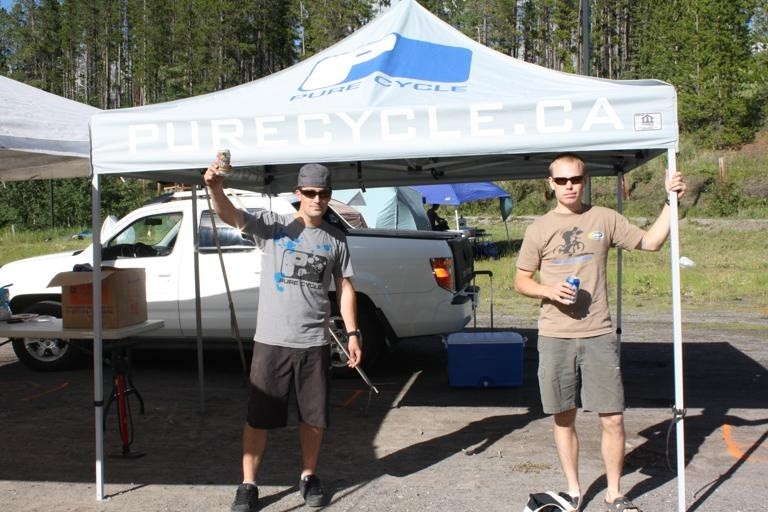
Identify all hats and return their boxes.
[291,164,337,192]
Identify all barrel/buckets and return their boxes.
[458,215,466,230]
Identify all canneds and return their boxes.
[216,149,230,177]
[565,276,580,301]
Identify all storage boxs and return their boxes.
[45,265,148,332]
[439,328,529,390]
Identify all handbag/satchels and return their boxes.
[522,490,577,512]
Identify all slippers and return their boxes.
[603,495,641,511]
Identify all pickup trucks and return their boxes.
[1,194,482,372]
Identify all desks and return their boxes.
[0,314,166,436]
[434,226,492,262]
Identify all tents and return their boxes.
[277,175,433,232]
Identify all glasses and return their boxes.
[552,175,585,185]
[297,187,333,199]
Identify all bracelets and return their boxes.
[345,329,361,339]
[665,198,681,207]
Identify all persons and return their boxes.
[458,215,466,226]
[515,151,691,512]
[427,204,449,230]
[202,151,364,512]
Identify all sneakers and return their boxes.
[298,474,324,508]
[231,481,259,512]
[567,491,583,510]
